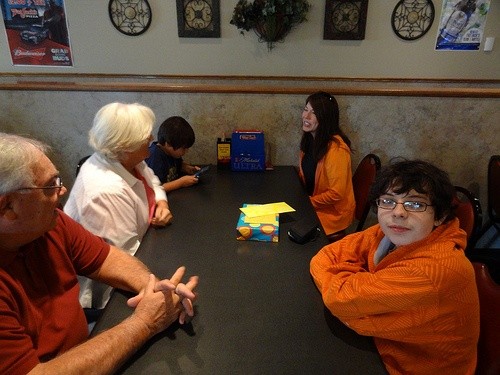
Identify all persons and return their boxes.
[309,157,480,375]
[63,102,173,336]
[300,91,358,242]
[146,116,201,188]
[0,133,198,375]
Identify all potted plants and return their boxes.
[229,0,310,48]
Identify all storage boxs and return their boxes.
[229,128,266,171]
[235,201,281,243]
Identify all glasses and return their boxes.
[19,177,62,196]
[376,197,434,212]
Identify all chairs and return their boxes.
[447,183,480,250]
[353,153,381,233]
[459,247,500,375]
[476,153,500,249]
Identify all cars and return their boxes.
[19,23,53,46]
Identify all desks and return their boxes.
[88,162,390,375]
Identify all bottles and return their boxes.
[220,132,226,142]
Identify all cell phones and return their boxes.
[194,165,212,178]
